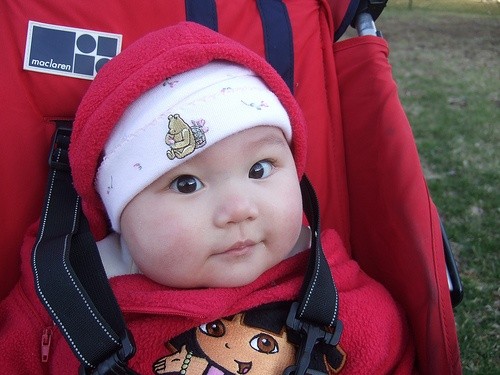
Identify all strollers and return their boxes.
[0,0,467,375]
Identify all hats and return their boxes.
[93,57,292,234]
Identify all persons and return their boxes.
[0,21,419,375]
